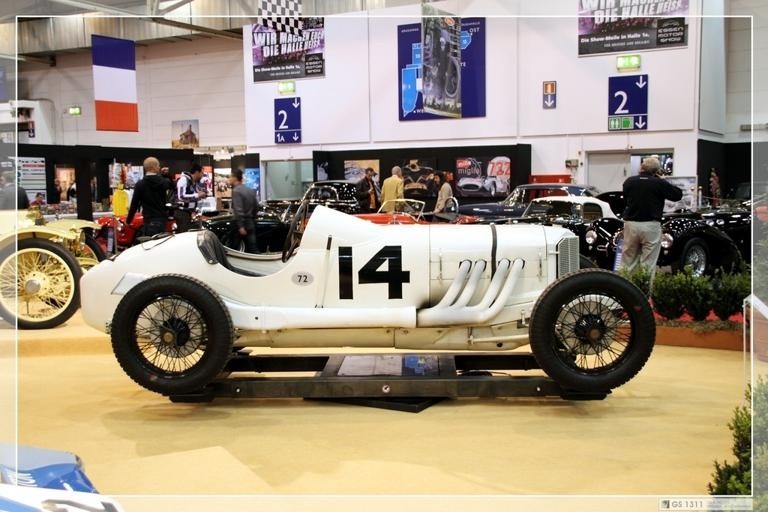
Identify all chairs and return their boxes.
[197,228,267,277]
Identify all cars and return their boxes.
[0,209,108,333]
[74,194,657,394]
[207,173,749,281]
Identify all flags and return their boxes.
[91,34,140,133]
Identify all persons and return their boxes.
[619,154,684,311]
[355,167,379,212]
[124,156,176,248]
[173,164,210,211]
[430,171,454,221]
[379,165,415,213]
[0,165,29,211]
[52,179,63,202]
[87,175,103,203]
[227,168,262,256]
[32,191,46,206]
